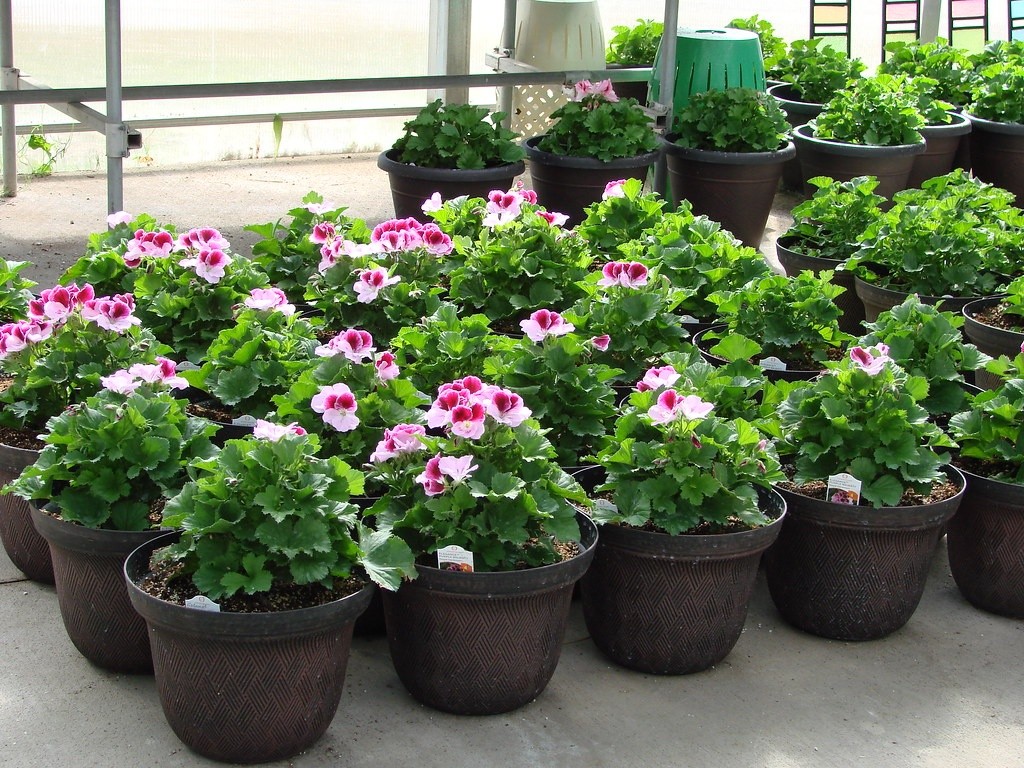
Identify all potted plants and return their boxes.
[377,16,1023,636]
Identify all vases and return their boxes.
[0,137,967,764]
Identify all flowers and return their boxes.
[0,80,948,577]
